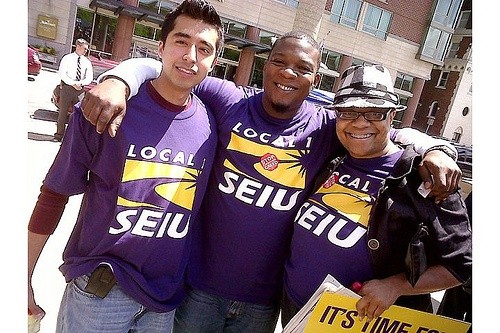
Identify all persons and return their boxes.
[52,38,93,139]
[279,61,469,333]
[29,2,221,332]
[87,29,462,332]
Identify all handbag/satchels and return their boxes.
[407,222,437,288]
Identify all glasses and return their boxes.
[334,108,393,121]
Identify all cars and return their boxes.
[28,44,42,75]
[53,53,120,112]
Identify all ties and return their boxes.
[76,57,81,81]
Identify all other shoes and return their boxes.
[53,135,64,142]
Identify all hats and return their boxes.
[323,64,408,111]
